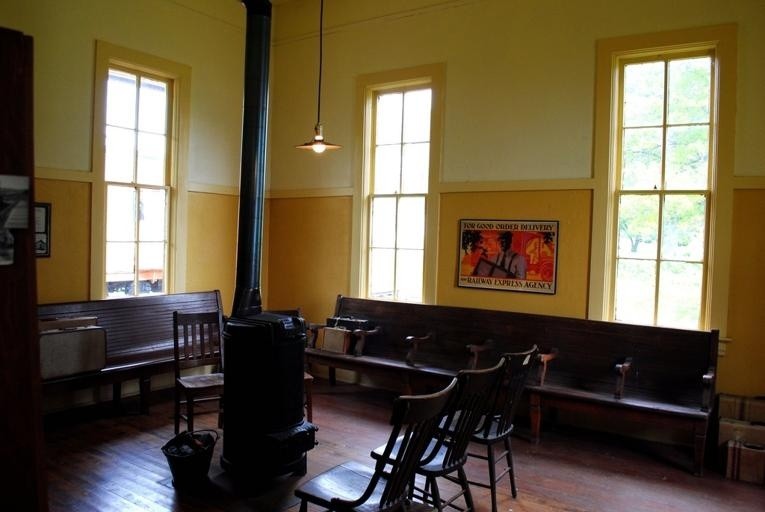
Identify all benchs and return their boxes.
[34,290,228,424]
[306,290,723,476]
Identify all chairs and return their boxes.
[265,305,315,425]
[294,343,540,510]
[170,308,228,435]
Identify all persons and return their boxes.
[490,232,528,279]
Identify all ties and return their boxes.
[500,254,506,269]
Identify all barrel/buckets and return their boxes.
[161,428,220,496]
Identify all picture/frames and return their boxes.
[34,202,52,257]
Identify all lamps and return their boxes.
[295,0,343,153]
